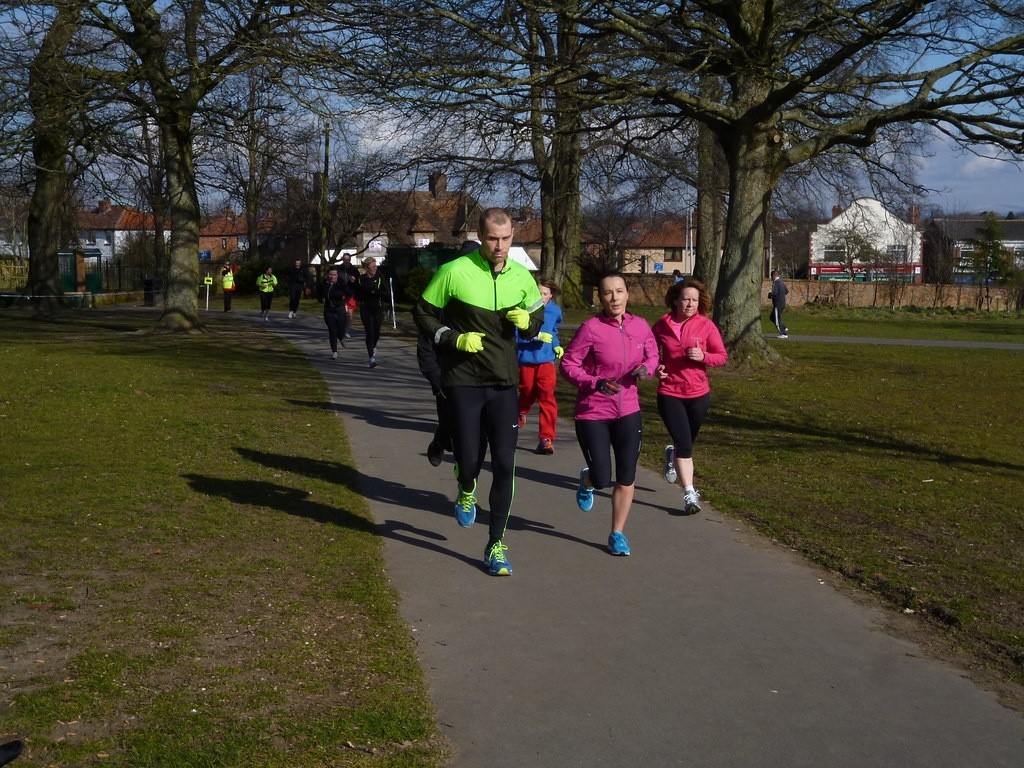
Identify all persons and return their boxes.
[336,252,359,339]
[670,269,682,283]
[257,266,278,321]
[316,266,349,360]
[412,207,545,574]
[650,278,728,514]
[559,272,659,556]
[768,269,790,339]
[218,261,235,314]
[353,257,390,369]
[282,258,312,318]
[515,274,564,455]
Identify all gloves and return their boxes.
[630,364,648,381]
[456,330,486,353]
[596,375,620,396]
[505,306,530,330]
[555,346,564,359]
[540,331,552,344]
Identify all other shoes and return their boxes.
[332,352,338,359]
[340,339,347,347]
[265,317,269,322]
[428,428,444,466]
[369,357,377,367]
[373,347,377,356]
[287,312,296,318]
[345,333,350,338]
[226,309,233,313]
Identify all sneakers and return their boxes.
[518,414,526,429]
[576,467,595,512]
[683,490,701,515]
[537,439,554,455]
[454,479,476,528]
[484,540,512,576]
[607,531,630,556]
[664,445,677,484]
